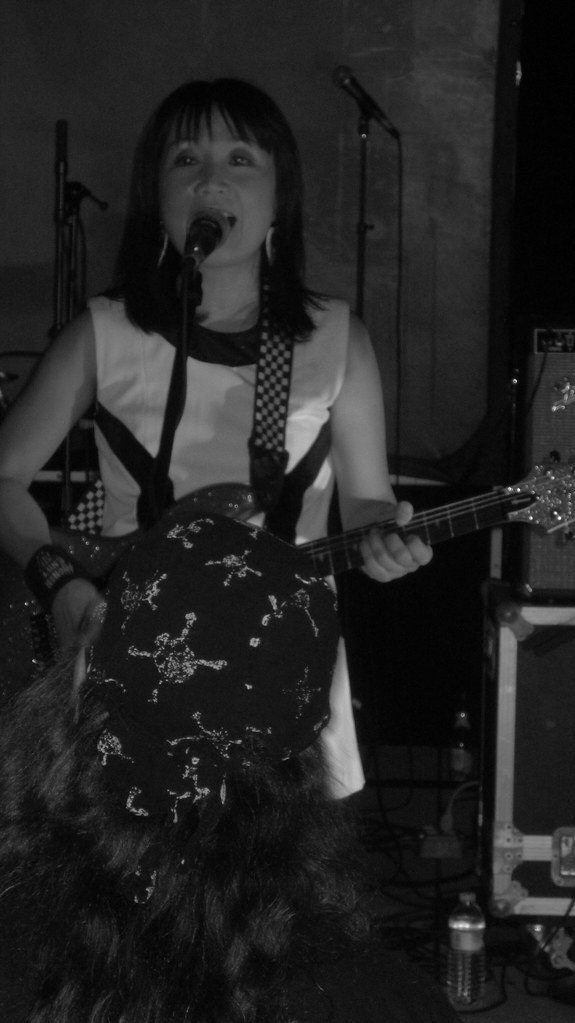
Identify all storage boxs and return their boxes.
[484,601,575,922]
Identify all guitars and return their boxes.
[0,443,574,719]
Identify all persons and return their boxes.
[0,510,460,1021]
[0,77,436,802]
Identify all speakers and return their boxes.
[513,323,575,606]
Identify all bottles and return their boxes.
[446,892,487,1007]
[448,691,474,785]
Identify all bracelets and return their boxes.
[23,541,85,612]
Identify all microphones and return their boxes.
[334,64,400,141]
[182,207,231,269]
